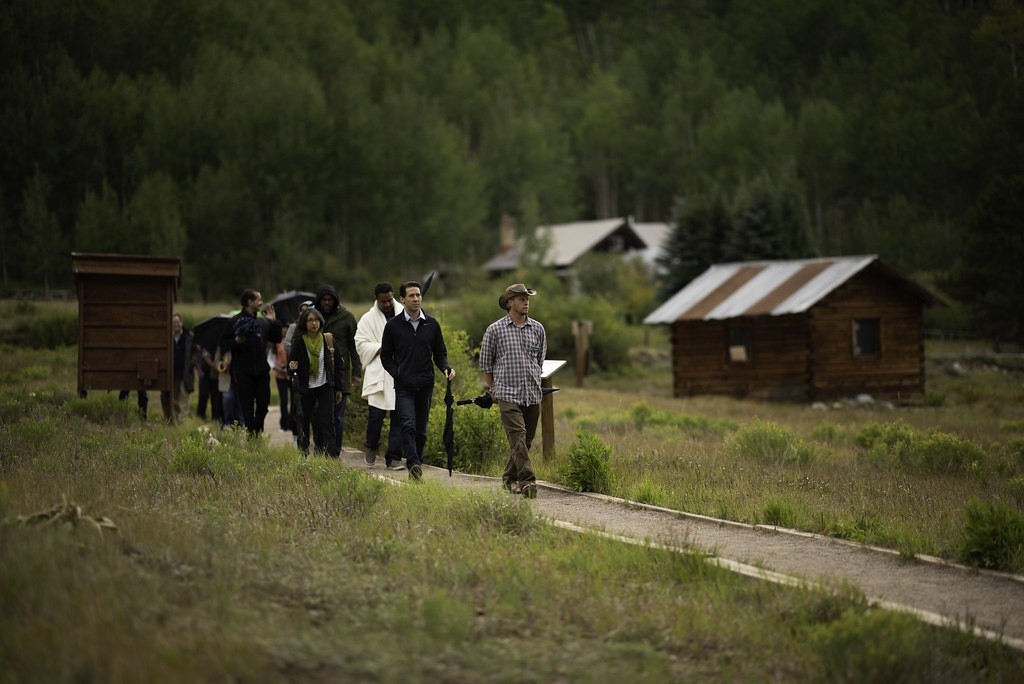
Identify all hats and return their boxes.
[498,284,537,310]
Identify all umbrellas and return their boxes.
[261,290,317,326]
[444,369,454,478]
[457,388,561,408]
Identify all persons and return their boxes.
[354,284,404,471]
[173,284,358,459]
[380,281,455,484]
[479,283,548,498]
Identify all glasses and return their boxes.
[306,316,320,323]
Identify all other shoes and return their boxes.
[409,465,422,481]
[502,480,521,493]
[387,460,405,470]
[522,483,537,499]
[364,447,376,465]
